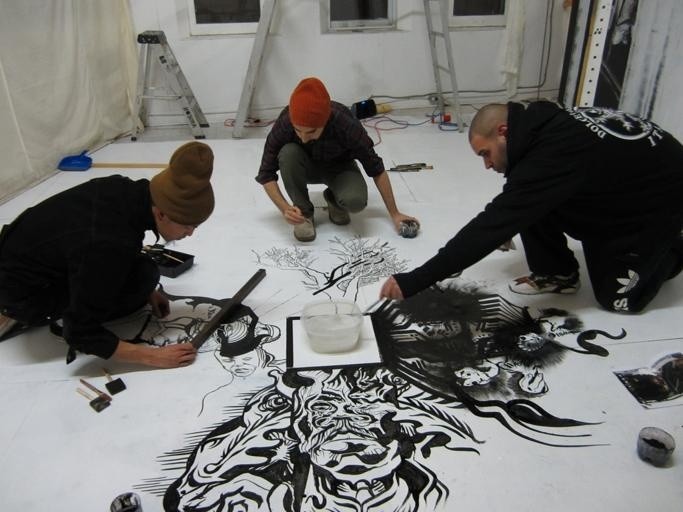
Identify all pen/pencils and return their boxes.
[103,368,126,396]
[77,388,111,412]
[289,206,310,224]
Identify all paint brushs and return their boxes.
[386,163,433,172]
[80,379,112,401]
[361,297,382,316]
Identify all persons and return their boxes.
[0,176,214,370]
[378,98,682,318]
[254,76,421,242]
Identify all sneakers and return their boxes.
[323,188,350,225]
[294,217,316,242]
[508,272,580,295]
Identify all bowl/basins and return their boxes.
[304,300,363,353]
[637,426,674,466]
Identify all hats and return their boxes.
[290,78,331,128]
[151,142,214,226]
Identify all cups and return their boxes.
[109,492,141,511]
[399,218,419,237]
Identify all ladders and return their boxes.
[131,31,210,141]
[232,0,277,138]
[422,1,463,131]
